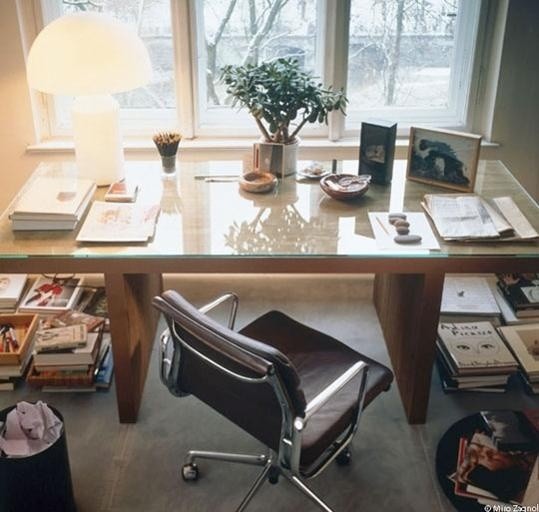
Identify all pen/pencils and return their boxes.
[195,175,241,182]
[0,324,19,353]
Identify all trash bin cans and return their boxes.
[0,402,76,510]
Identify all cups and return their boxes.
[163,175,178,198]
[158,156,178,174]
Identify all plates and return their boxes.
[298,168,333,179]
[294,177,321,184]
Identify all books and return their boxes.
[9,171,162,249]
[431,267,538,511]
[0,270,113,397]
[424,187,539,248]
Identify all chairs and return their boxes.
[153,290,395,512]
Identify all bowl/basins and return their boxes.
[318,196,373,217]
[318,173,370,201]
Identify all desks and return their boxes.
[3,161,537,425]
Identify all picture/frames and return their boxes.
[406,126,482,194]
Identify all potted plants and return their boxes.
[221,60,348,175]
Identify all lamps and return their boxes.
[25,11,151,186]
